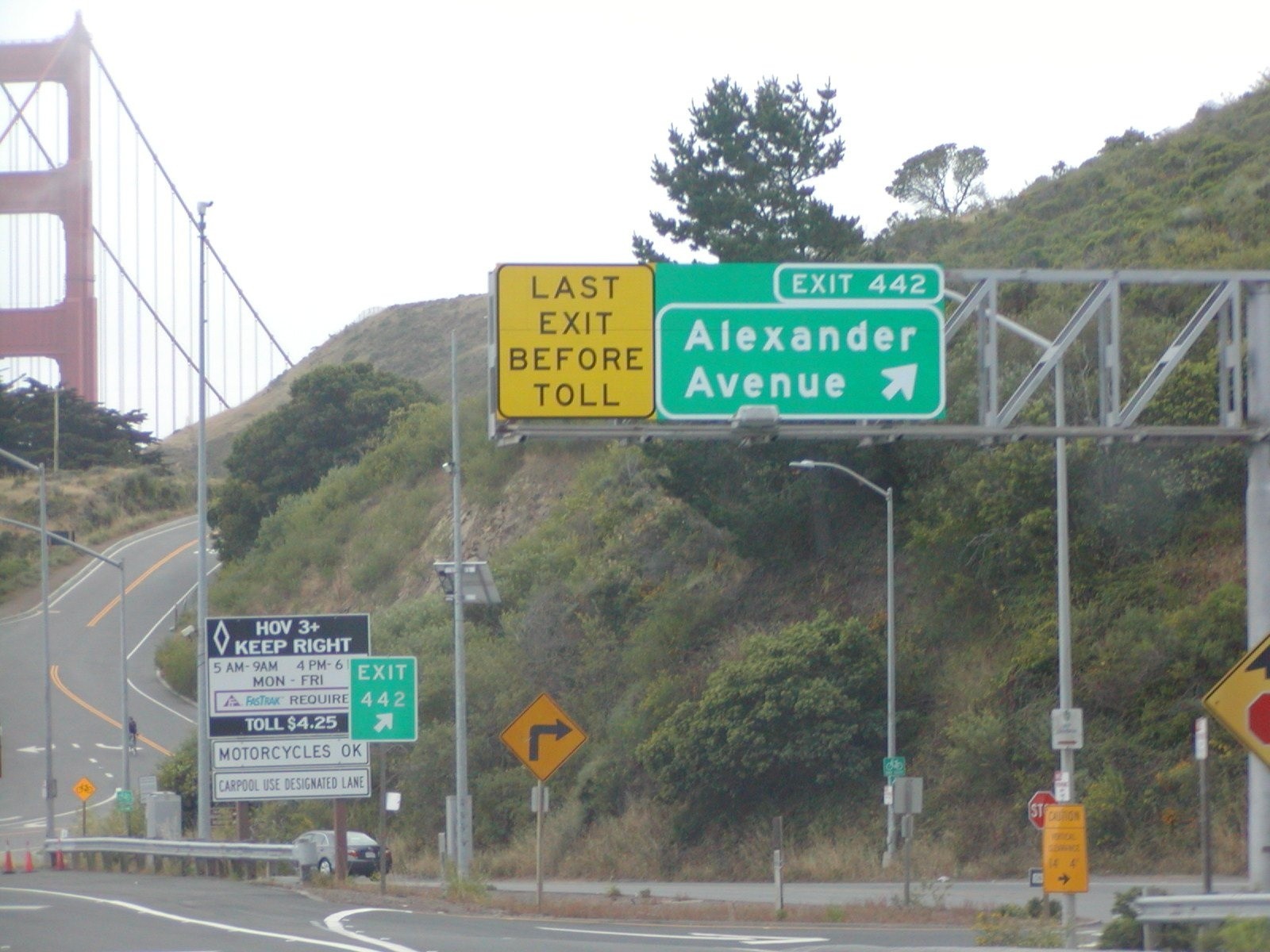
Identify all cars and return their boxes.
[290,829,393,882]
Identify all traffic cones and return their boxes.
[22,840,37,873]
[52,851,67,873]
[2,840,18,874]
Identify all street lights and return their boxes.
[787,459,899,870]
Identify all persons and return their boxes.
[128,716,138,747]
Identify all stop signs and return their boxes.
[1028,791,1058,830]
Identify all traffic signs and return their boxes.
[348,655,419,743]
[493,262,947,421]
